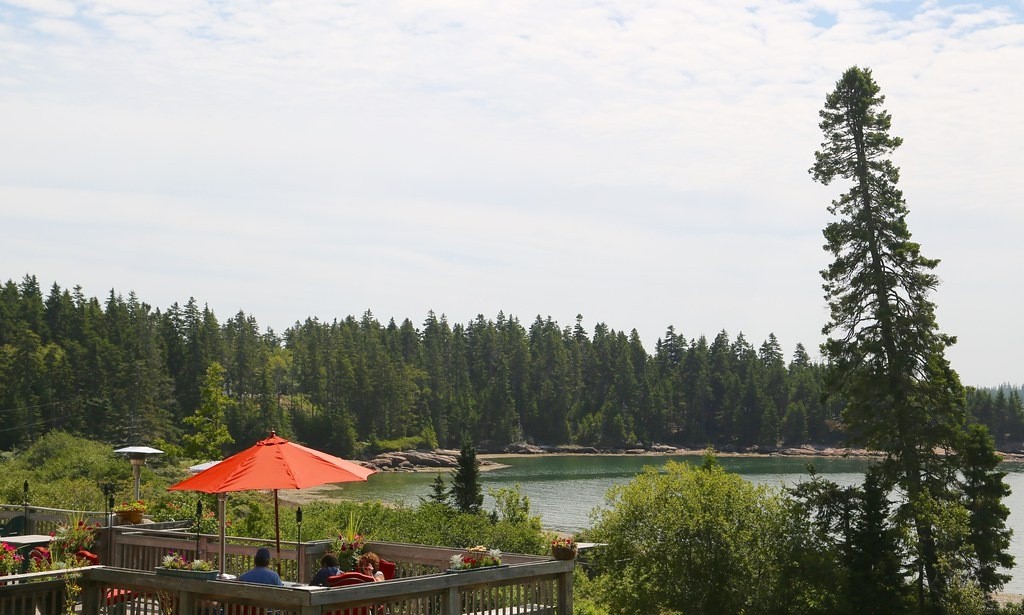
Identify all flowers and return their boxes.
[552,537,576,548]
[162,550,216,571]
[0,542,23,573]
[45,506,97,555]
[328,509,382,571]
[449,545,503,569]
[111,500,147,512]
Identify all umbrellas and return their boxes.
[167,429,376,579]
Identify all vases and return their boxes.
[551,547,576,560]
[447,563,509,573]
[154,567,219,580]
[0,573,8,587]
[114,511,145,525]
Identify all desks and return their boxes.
[576,543,611,550]
[0,534,58,583]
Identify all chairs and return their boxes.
[328,572,375,615]
[73,551,138,606]
[0,516,35,537]
[370,559,394,613]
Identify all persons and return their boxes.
[357,552,396,580]
[309,555,342,587]
[239,548,282,585]
